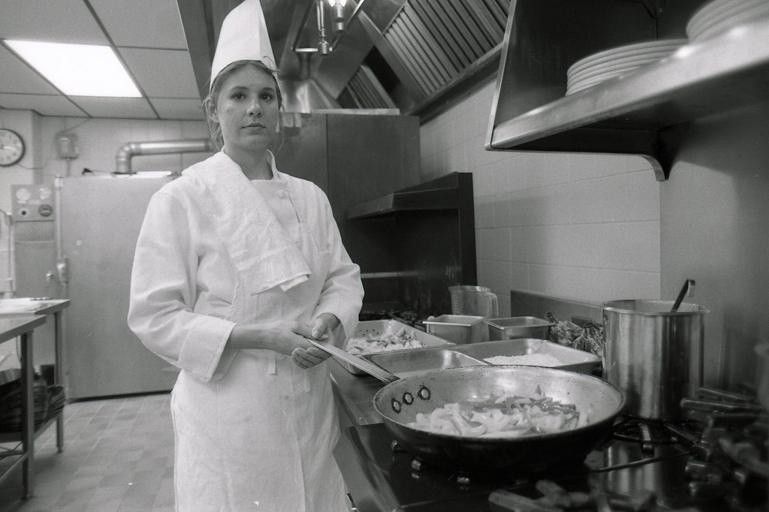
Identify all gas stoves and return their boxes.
[331,386,769,512]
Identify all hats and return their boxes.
[209,0,283,105]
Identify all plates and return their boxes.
[564,0,768,100]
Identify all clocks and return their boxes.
[0,129,24,166]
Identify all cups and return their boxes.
[450,284,502,319]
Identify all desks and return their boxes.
[1,298,70,466]
[1,314,48,500]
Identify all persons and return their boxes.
[127,1,367,512]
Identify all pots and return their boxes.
[603,298,712,422]
[302,336,628,466]
